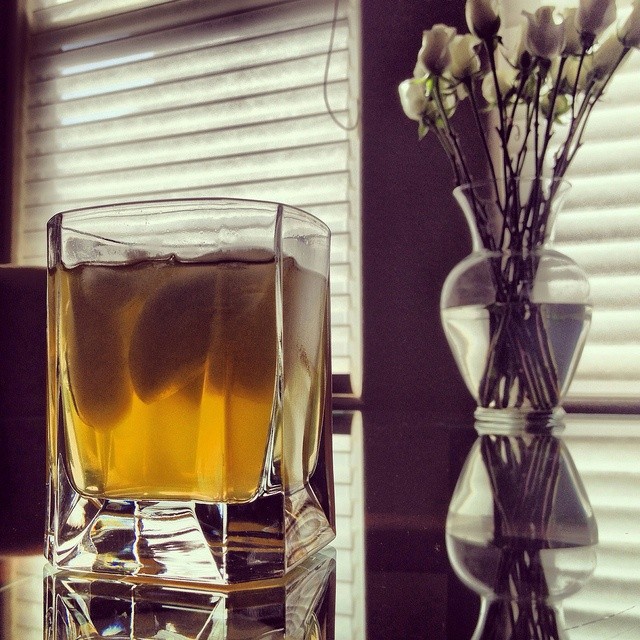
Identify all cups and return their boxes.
[43,198,336,593]
[42,547,336,639]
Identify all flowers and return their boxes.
[398,0,640,410]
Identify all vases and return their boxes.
[445,422,600,639]
[441,178,593,424]
